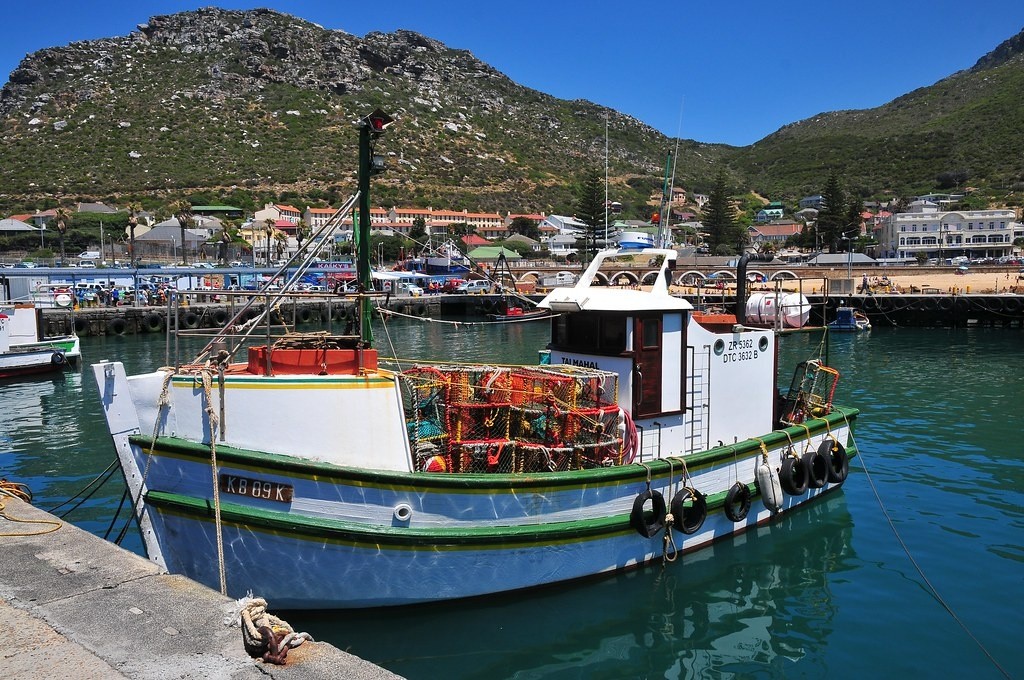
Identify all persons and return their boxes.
[858,272,871,295]
[68,277,472,310]
[591,279,642,291]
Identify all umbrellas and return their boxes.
[247,275,269,290]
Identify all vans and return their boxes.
[77,251,101,259]
[443,278,491,294]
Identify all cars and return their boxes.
[396,283,425,296]
[4,260,95,268]
[929,255,1024,263]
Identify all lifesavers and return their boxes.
[108,318,130,336]
[632,490,667,540]
[682,296,1024,313]
[804,451,830,489]
[296,308,313,324]
[241,309,259,324]
[52,353,65,365]
[211,310,228,327]
[271,309,284,324]
[818,439,850,483]
[725,482,751,522]
[320,298,532,323]
[671,487,708,535]
[75,318,90,336]
[165,316,181,329]
[142,312,165,333]
[181,312,201,329]
[780,458,809,496]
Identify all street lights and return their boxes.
[841,237,858,279]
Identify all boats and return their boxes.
[485,298,549,321]
[0,284,81,378]
[92,107,859,617]
[827,307,872,332]
